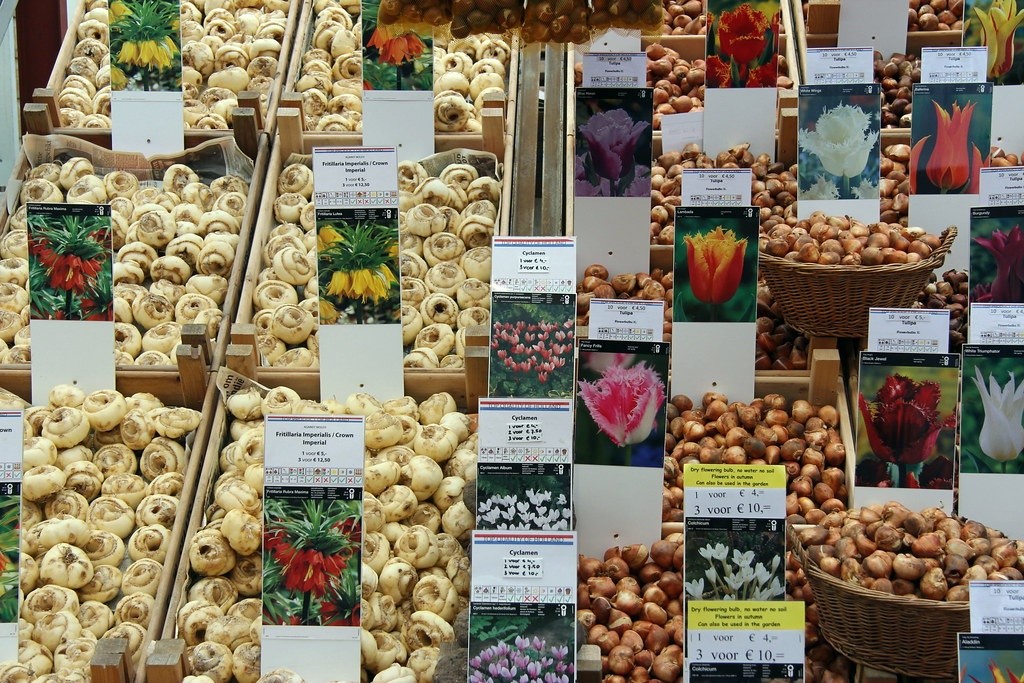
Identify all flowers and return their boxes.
[675,223,757,322]
[491,318,574,384]
[361,1,427,92]
[971,224,1024,303]
[315,219,401,324]
[263,485,361,627]
[469,634,574,683]
[962,0,1024,85]
[798,100,879,200]
[108,0,184,91]
[964,364,1024,474]
[707,3,777,87]
[576,351,666,466]
[858,373,957,490]
[685,542,785,601]
[477,487,571,530]
[910,99,990,194]
[27,215,114,321]
[576,108,650,197]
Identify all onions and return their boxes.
[575,391,857,683]
[799,500,1024,601]
[378,0,663,44]
[575,0,1024,374]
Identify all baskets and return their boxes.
[788,525,971,677]
[758,225,957,338]
